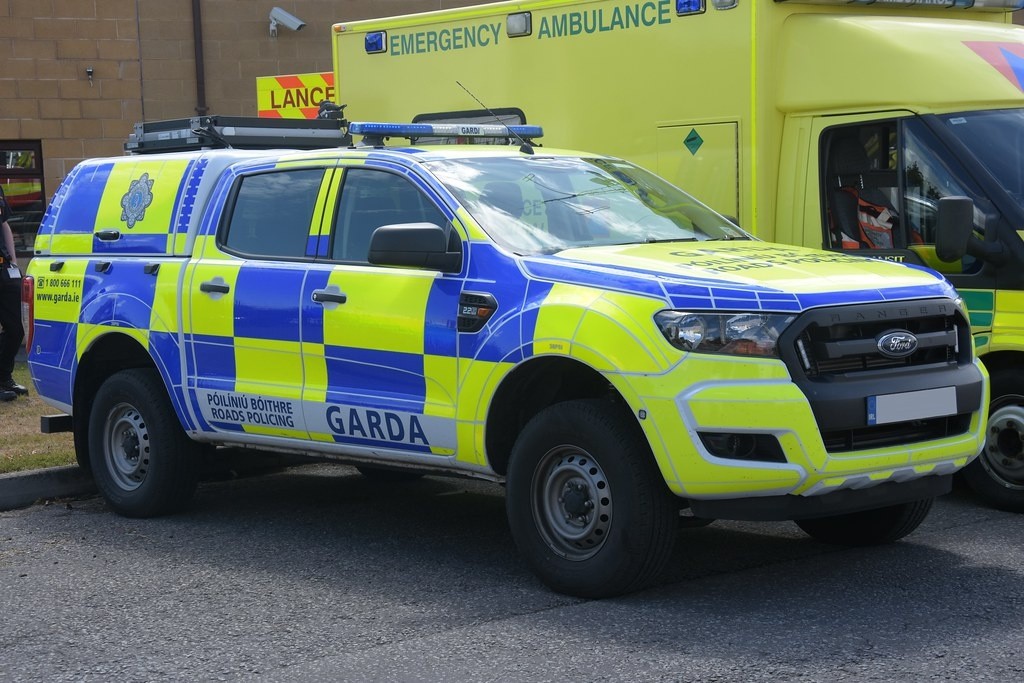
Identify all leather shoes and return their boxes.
[0,388,16,400]
[0,379,28,394]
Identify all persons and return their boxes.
[0,183,29,402]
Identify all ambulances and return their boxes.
[331,0,1024,513]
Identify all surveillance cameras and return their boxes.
[268,7,304,36]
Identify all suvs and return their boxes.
[21,100,990,600]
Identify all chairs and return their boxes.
[835,172,921,253]
